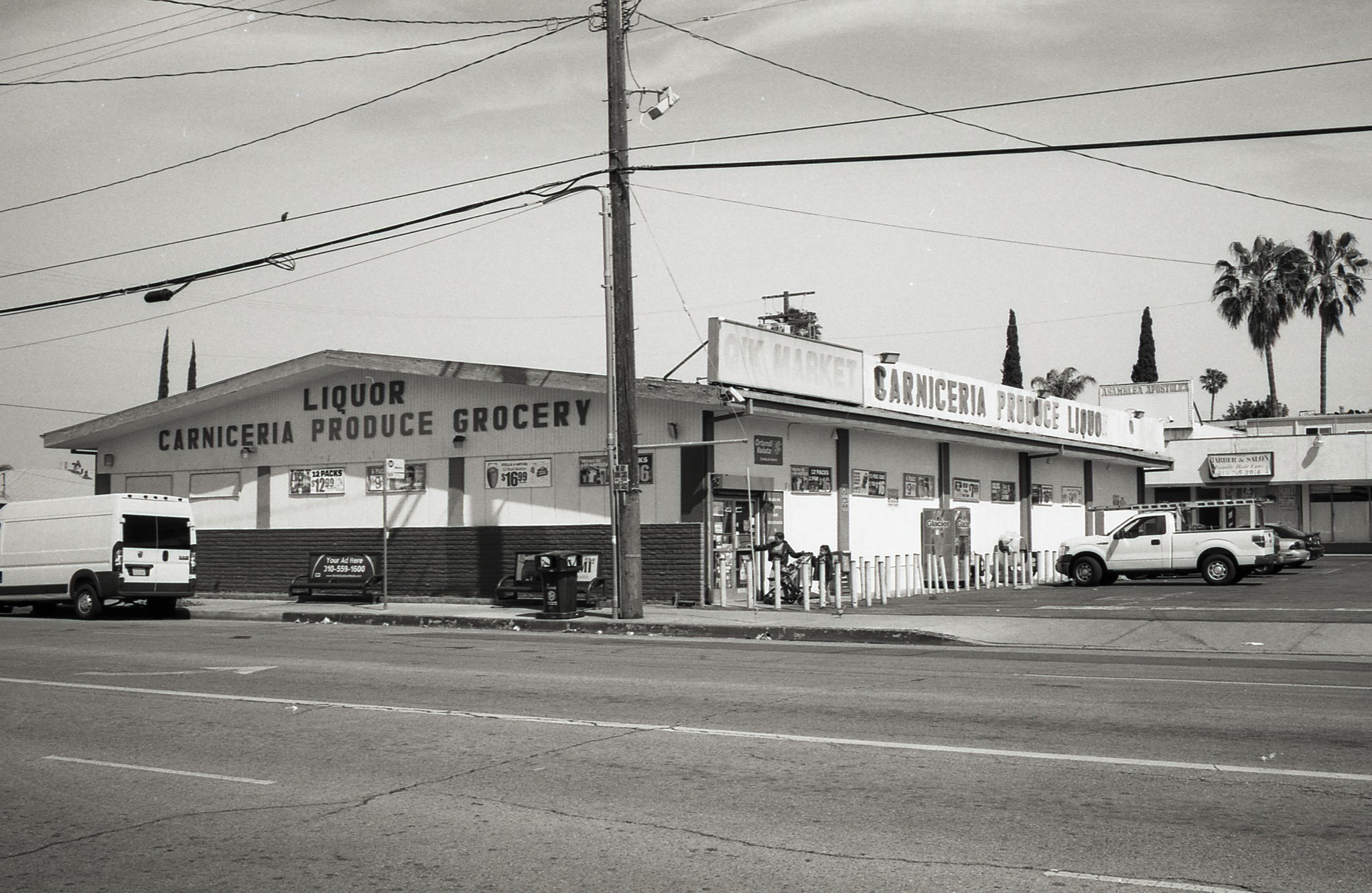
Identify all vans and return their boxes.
[0,493,198,622]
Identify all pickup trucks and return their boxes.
[1054,511,1281,588]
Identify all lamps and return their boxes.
[1046,457,1052,464]
[1037,390,1051,399]
[1313,434,1323,448]
[667,422,678,439]
[240,446,258,460]
[1132,411,1145,419]
[830,427,839,439]
[453,435,466,448]
[1106,463,1112,471]
[104,454,114,466]
[874,352,900,363]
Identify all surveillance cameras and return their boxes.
[1168,416,1174,423]
[648,93,679,120]
[726,387,745,402]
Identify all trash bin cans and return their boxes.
[535,551,584,618]
[829,551,852,596]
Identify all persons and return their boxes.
[811,545,833,606]
[745,532,805,605]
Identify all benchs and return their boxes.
[288,551,384,605]
[494,551,608,610]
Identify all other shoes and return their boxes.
[816,600,820,604]
[826,601,831,606]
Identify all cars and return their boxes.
[1236,522,1325,567]
[1253,536,1311,575]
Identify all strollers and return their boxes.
[764,552,813,605]
